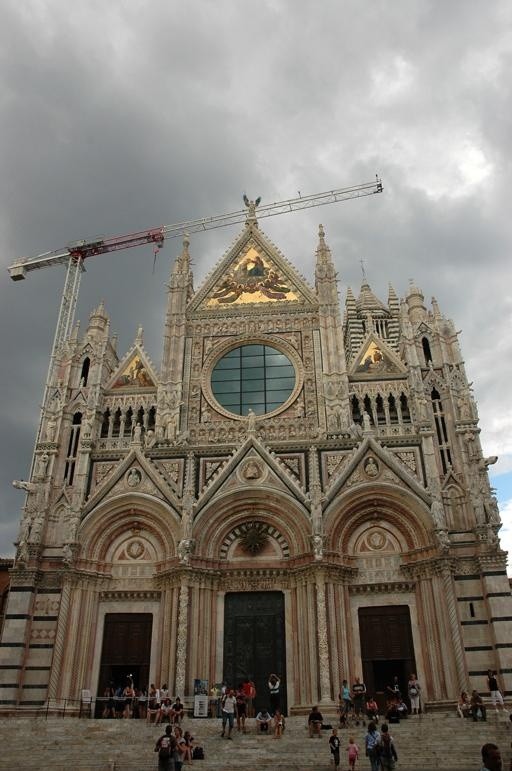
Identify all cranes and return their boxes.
[5,173,384,570]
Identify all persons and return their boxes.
[100,681,185,725]
[328,726,342,766]
[154,725,184,771]
[345,737,360,771]
[479,742,503,771]
[169,726,186,771]
[182,730,194,764]
[365,722,381,771]
[376,723,399,771]
[268,673,281,716]
[485,669,509,713]
[385,675,422,722]
[193,684,208,697]
[308,706,325,738]
[210,676,255,740]
[337,676,378,726]
[256,709,285,739]
[457,690,488,723]
